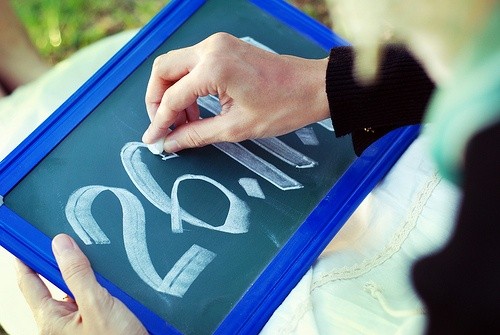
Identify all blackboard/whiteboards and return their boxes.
[0,0,428,335]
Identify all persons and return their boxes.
[0,7,499,335]
